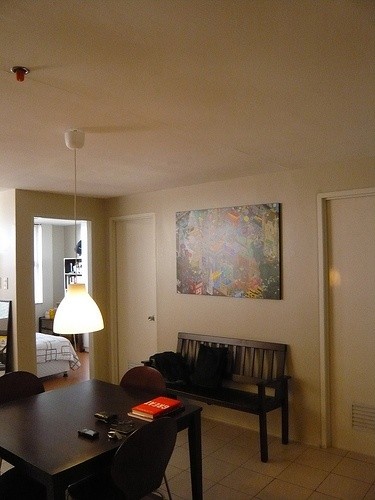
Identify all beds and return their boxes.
[36,331,82,378]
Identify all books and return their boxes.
[65,275,76,289]
[127,395,183,422]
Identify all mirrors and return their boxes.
[0,300,12,376]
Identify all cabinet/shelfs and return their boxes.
[39,316,73,352]
[64,258,89,353]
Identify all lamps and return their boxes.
[53,128,105,335]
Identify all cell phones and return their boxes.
[95,412,117,419]
[110,425,134,434]
[78,429,99,439]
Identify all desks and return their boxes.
[0,379,203,500]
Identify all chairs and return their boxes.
[0,371,46,404]
[120,367,167,398]
[64,416,177,500]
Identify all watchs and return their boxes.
[107,431,122,440]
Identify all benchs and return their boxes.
[140,333,292,462]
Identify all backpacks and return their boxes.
[189,344,235,397]
[149,351,193,391]
